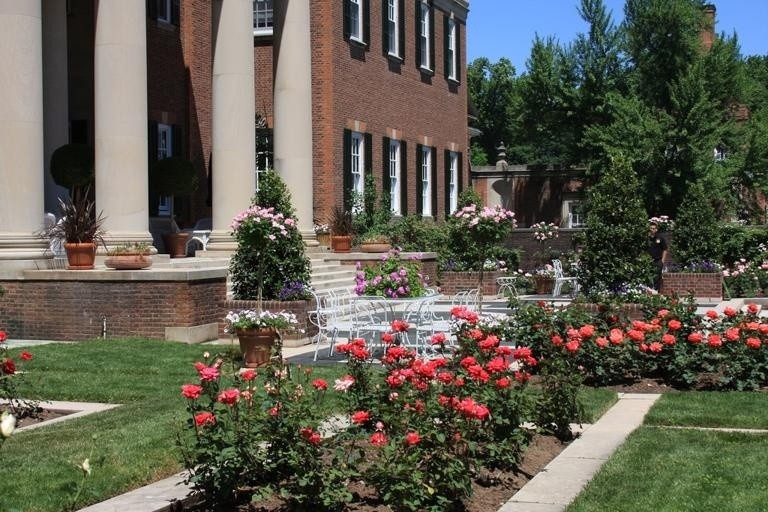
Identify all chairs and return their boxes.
[306,287,479,362]
[552,258,579,298]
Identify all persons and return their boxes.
[645,221,668,293]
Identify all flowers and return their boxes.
[220,306,305,348]
[352,244,431,297]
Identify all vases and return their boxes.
[236,324,276,369]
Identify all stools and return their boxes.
[496,276,518,299]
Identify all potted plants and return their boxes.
[35,182,156,270]
